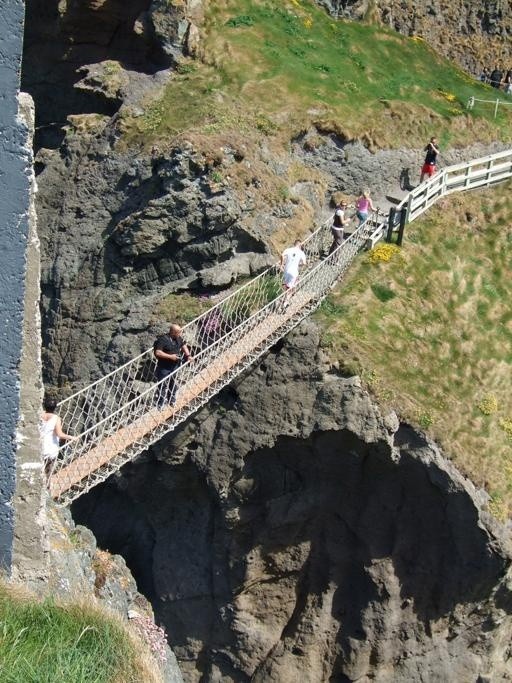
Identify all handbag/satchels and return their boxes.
[185,354,191,358]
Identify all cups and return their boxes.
[341,204,347,207]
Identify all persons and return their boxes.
[356,188,384,228]
[330,200,351,253]
[479,63,512,93]
[42,398,77,489]
[277,239,307,315]
[420,137,440,183]
[154,324,194,412]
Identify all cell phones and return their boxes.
[178,355,183,359]
[428,144,431,147]
